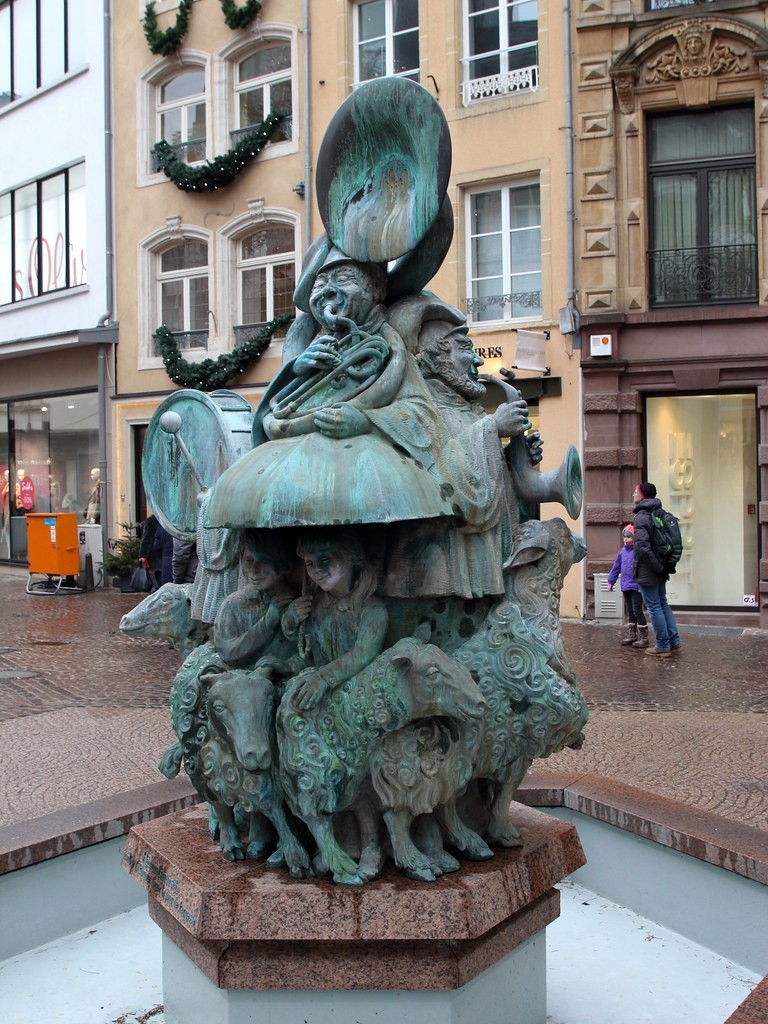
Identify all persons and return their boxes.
[2,470,29,560]
[606,524,649,648]
[632,482,683,658]
[85,469,101,524]
[138,514,199,586]
[160,248,586,883]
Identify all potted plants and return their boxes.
[96,520,146,591]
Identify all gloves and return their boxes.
[663,570,670,581]
[664,561,677,574]
[607,583,613,591]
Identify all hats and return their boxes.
[638,483,657,499]
[622,524,634,538]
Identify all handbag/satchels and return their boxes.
[130,560,153,593]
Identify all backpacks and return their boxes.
[651,508,683,563]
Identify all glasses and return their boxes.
[623,535,631,538]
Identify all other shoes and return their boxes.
[670,645,683,653]
[645,647,670,657]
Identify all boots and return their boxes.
[621,623,638,645]
[632,624,650,647]
